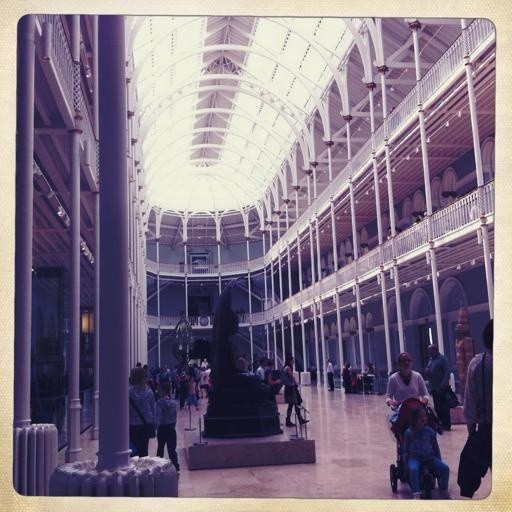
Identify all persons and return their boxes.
[326,358,334,391]
[341,360,376,395]
[178,307,246,323]
[385,318,493,500]
[129,353,310,474]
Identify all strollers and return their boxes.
[385,397,442,496]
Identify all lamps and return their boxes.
[80,305,94,343]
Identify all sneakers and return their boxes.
[284,415,310,427]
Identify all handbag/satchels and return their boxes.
[430,385,461,414]
[142,422,157,439]
